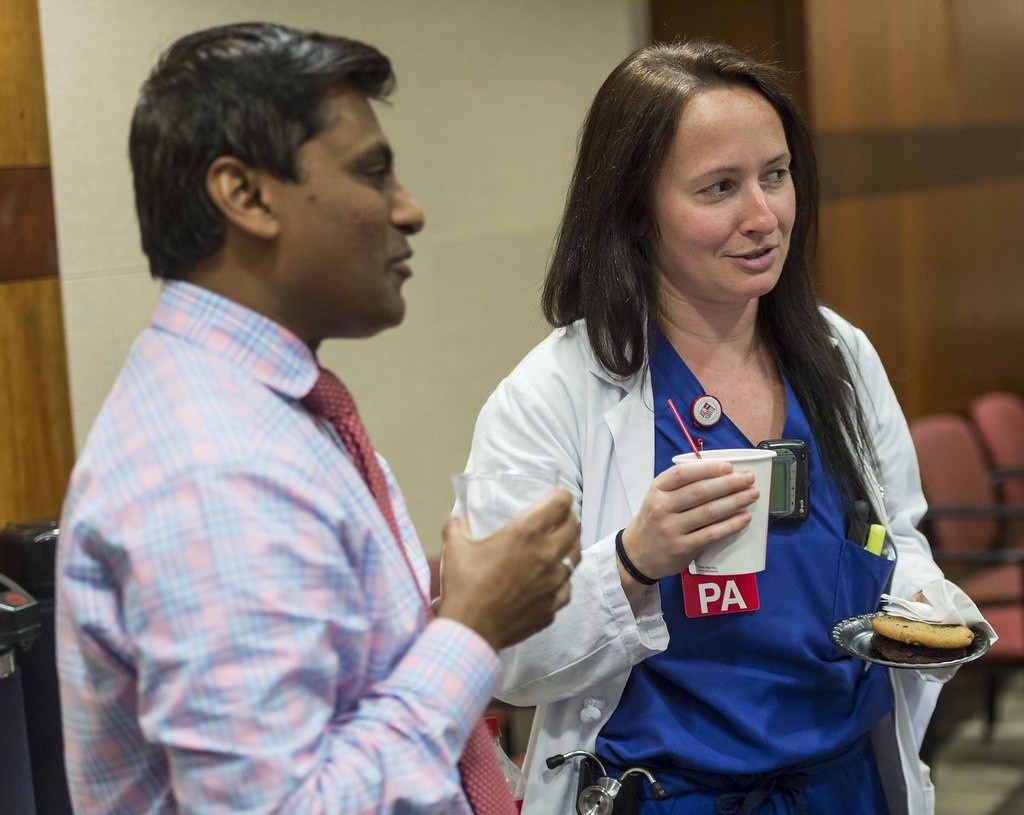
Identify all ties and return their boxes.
[300,366,518,815]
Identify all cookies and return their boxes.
[871,614,975,648]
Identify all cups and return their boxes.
[452,469,564,545]
[672,448,776,576]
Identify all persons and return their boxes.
[441,39,999,815]
[55,23,583,814]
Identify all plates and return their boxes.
[834,612,989,669]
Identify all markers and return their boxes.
[846,496,888,556]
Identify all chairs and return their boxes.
[909,392,1024,749]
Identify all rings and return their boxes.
[561,556,575,573]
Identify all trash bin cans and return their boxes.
[0,520,75,815]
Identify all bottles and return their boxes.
[484,716,527,815]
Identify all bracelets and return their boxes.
[616,528,662,585]
